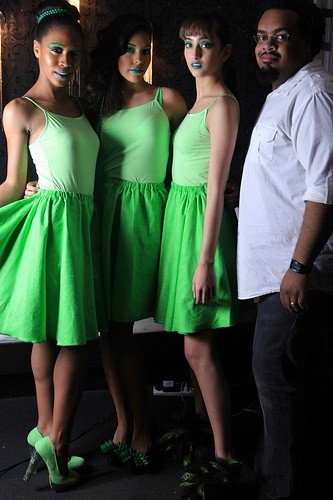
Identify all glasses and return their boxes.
[253,33,301,43]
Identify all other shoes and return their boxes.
[179,449,244,500]
[151,414,215,469]
[100,433,155,475]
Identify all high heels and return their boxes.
[22,423,86,491]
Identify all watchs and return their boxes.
[289,259,314,275]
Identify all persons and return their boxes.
[153,15,240,482]
[237,0,333,500]
[0,7,100,491]
[23,13,240,475]
